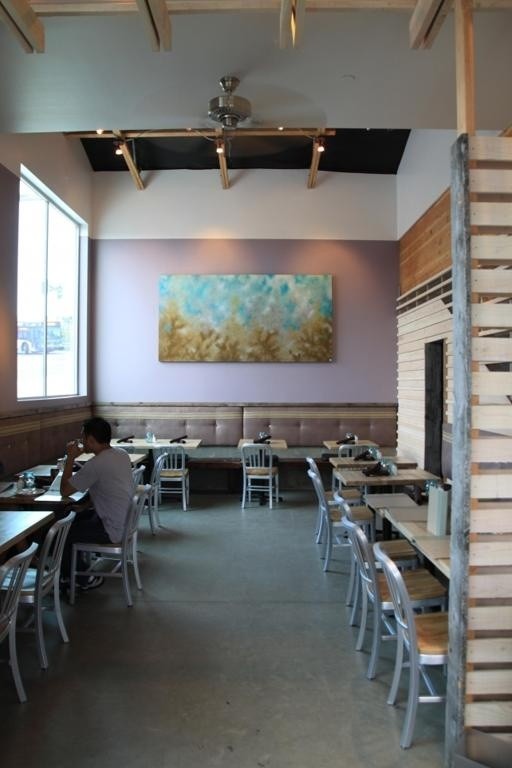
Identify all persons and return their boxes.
[25,416,137,600]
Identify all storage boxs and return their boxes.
[427,484,452,538]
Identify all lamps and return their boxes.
[113,140,124,156]
[314,137,326,151]
[214,139,224,153]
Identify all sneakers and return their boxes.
[60,571,79,584]
[82,573,104,590]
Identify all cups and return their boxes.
[257,432,268,439]
[379,458,397,475]
[23,472,35,490]
[76,438,83,449]
[146,432,154,443]
[345,432,358,443]
[425,479,438,502]
[368,448,381,460]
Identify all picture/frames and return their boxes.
[159,274,334,362]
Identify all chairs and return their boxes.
[334,492,418,627]
[241,443,279,510]
[135,454,168,537]
[306,457,362,534]
[69,483,152,607]
[132,465,146,485]
[341,516,447,681]
[308,469,375,572]
[373,542,448,750]
[0,542,39,703]
[154,446,189,511]
[0,511,77,673]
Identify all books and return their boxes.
[34,471,90,502]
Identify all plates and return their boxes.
[16,488,46,496]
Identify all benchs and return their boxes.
[121,448,397,494]
